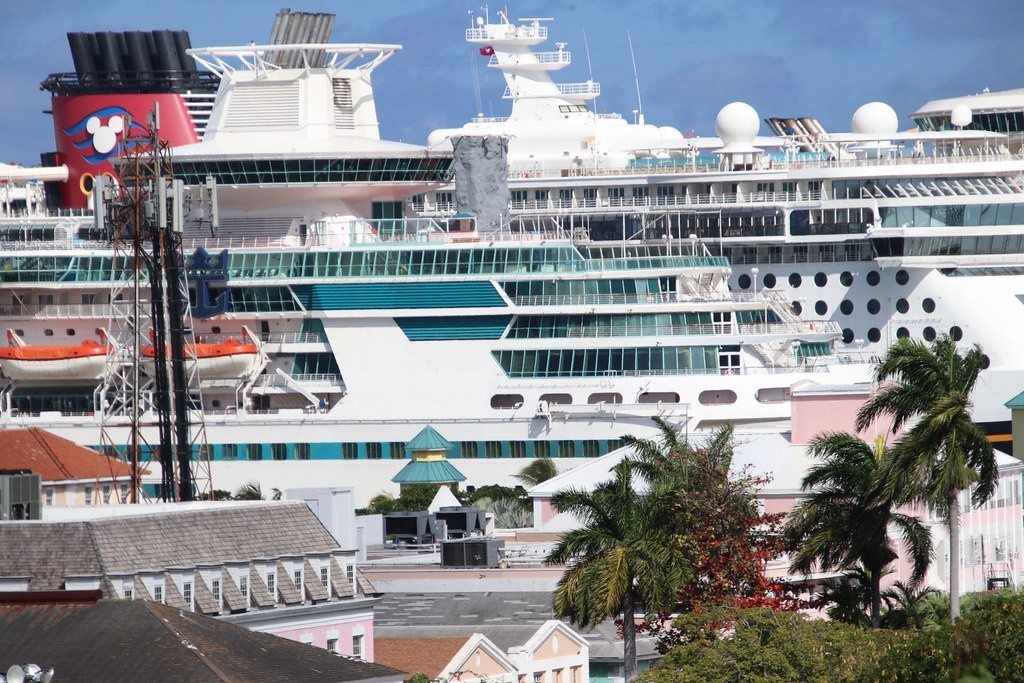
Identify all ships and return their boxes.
[0,0,1024,517]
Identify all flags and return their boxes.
[479,46,495,55]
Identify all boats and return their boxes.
[1,337,114,382]
[138,338,257,381]
